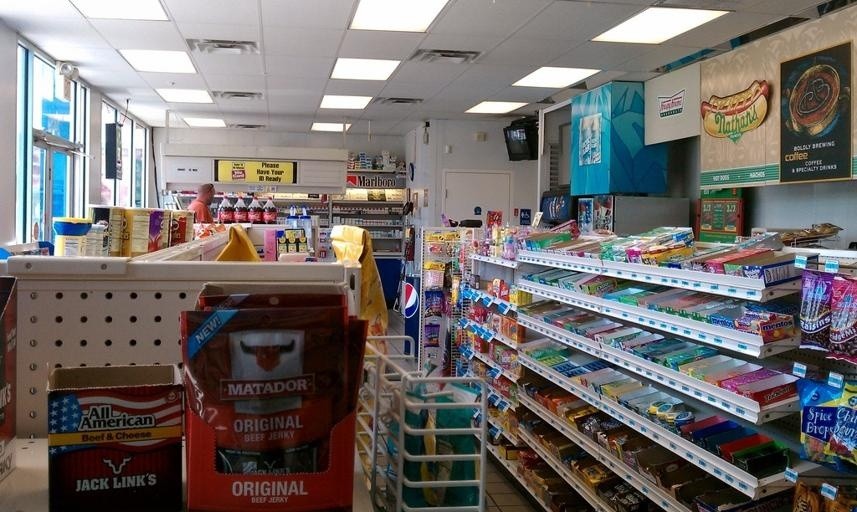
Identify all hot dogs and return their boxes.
[701,80,770,137]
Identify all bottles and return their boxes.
[217,196,277,224]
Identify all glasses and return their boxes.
[207,184,213,194]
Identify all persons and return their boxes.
[187,184,215,223]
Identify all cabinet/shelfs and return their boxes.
[173,194,857,512]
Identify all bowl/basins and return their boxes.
[52,217,91,235]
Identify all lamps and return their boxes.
[54,61,80,103]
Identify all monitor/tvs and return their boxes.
[540,190,577,225]
[503,117,538,161]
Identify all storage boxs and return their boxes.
[46,364,183,512]
